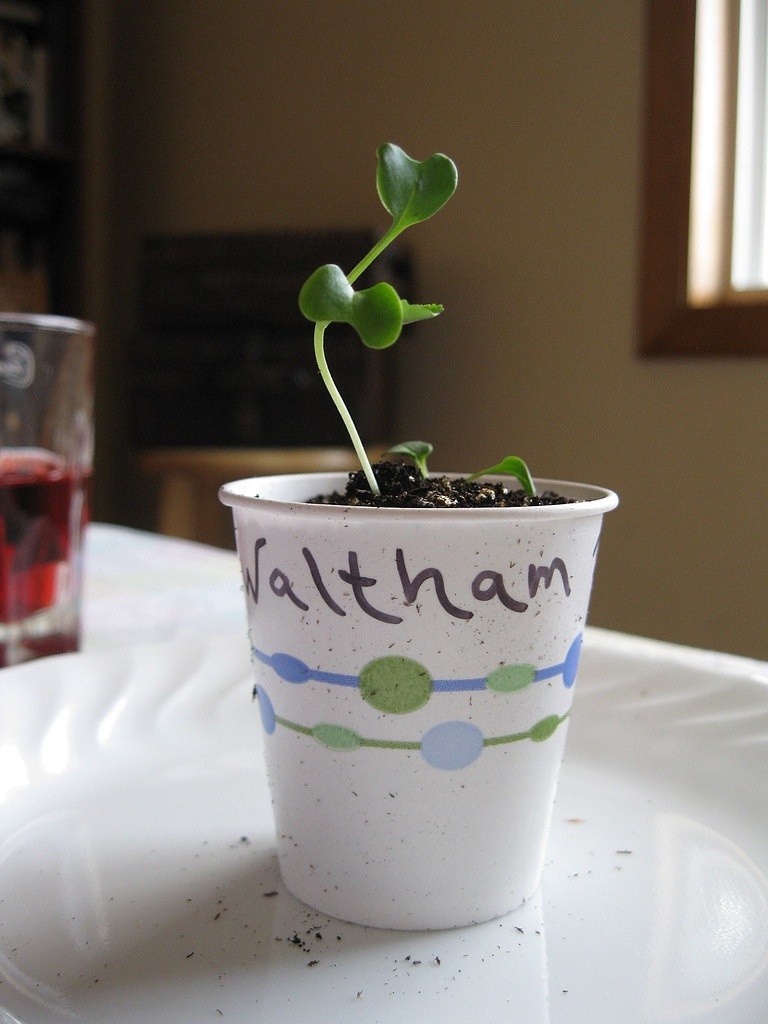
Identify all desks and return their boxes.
[139,446,402,549]
[0,523,768,1024]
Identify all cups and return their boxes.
[0,312,97,666]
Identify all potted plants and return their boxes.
[216,139,619,938]
